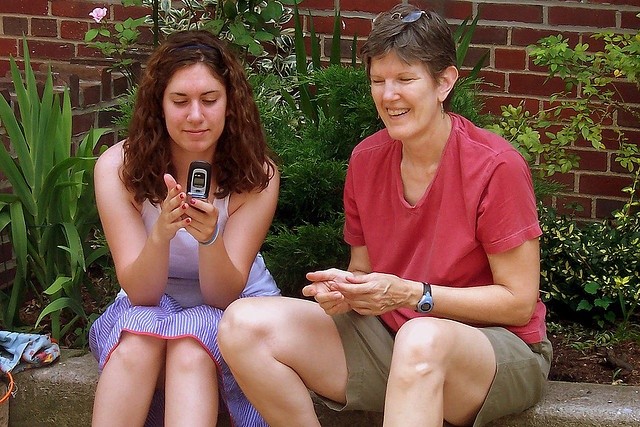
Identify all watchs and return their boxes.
[414,281,434,313]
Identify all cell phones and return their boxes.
[187,159,211,204]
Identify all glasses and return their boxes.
[373,10,429,25]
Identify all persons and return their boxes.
[88,29,281,426]
[216,6,554,426]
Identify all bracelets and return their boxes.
[198,224,220,245]
